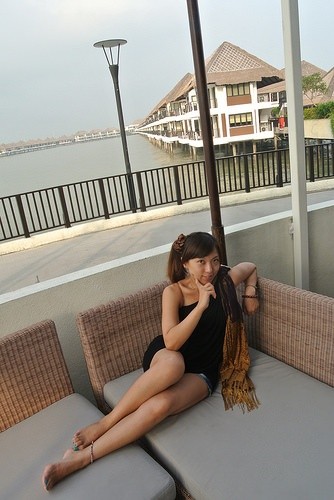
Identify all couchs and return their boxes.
[75,265,334,500]
[0,319,176,500]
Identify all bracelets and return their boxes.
[241,293,260,300]
[244,284,258,291]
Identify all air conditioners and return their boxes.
[260,126,269,132]
[259,94,268,102]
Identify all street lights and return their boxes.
[93,38,140,211]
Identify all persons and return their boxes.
[41,228,261,493]
